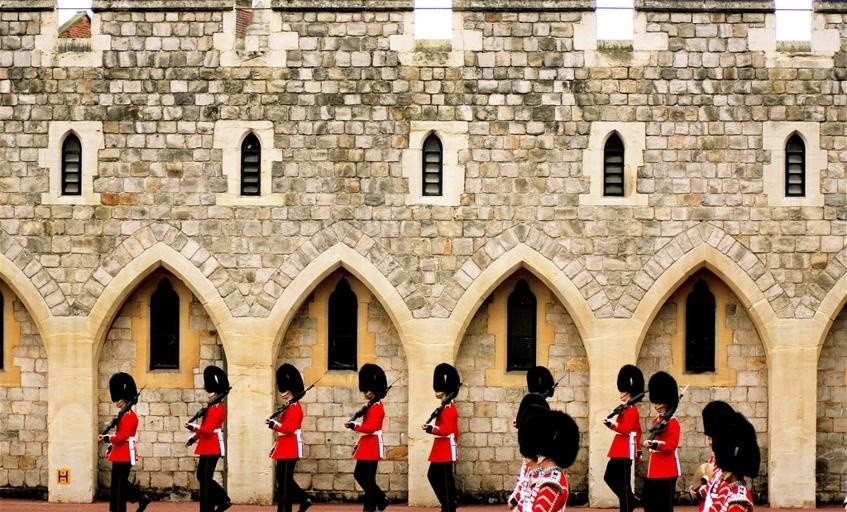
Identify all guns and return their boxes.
[605,391,648,421]
[648,384,690,445]
[347,377,401,422]
[185,387,232,425]
[542,375,567,399]
[267,376,322,422]
[425,383,463,423]
[98,384,148,438]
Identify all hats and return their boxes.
[616,364,645,402]
[358,363,387,399]
[526,366,555,397]
[515,394,580,469]
[433,363,460,399]
[702,401,736,438]
[276,363,305,400]
[109,372,138,406]
[711,411,761,479]
[204,365,230,396]
[648,370,679,409]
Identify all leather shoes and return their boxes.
[631,494,641,510]
[215,500,232,512]
[377,495,391,512]
[135,493,151,512]
[297,496,313,512]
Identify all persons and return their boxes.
[264,363,313,512]
[604,364,760,512]
[422,363,460,512]
[344,363,392,512]
[507,366,579,512]
[97,372,151,512]
[184,366,232,512]
